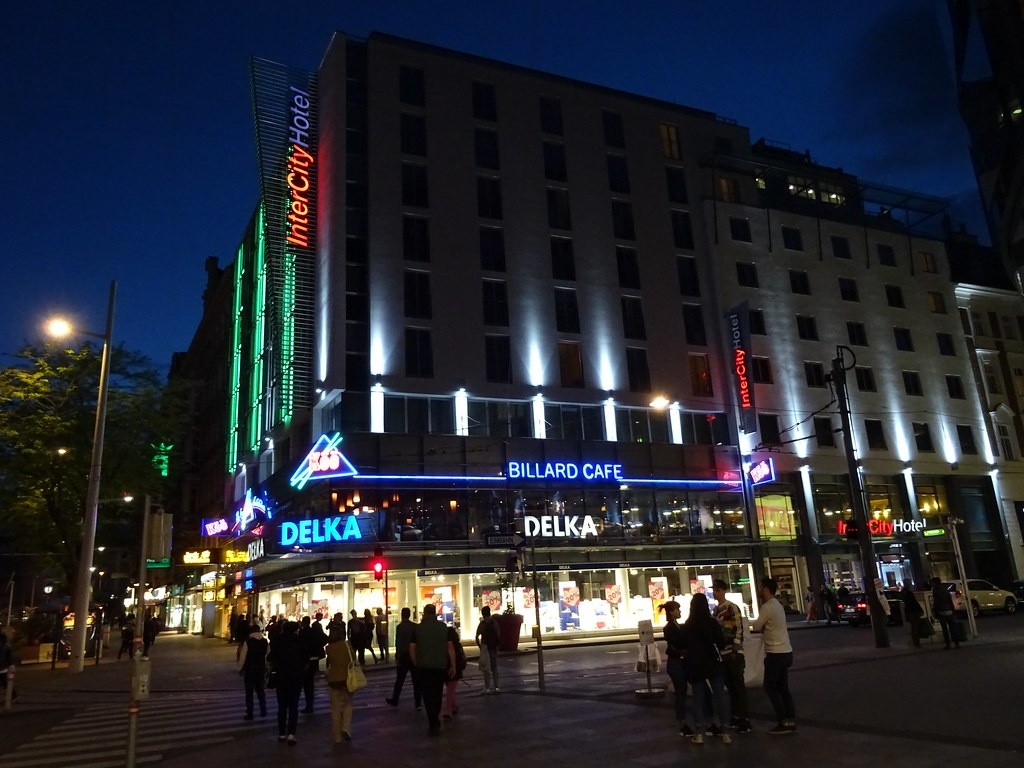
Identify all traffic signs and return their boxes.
[483,531,528,549]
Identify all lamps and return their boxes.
[951,461,959,471]
[239,456,245,466]
[315,380,325,393]
[457,378,466,392]
[673,394,680,405]
[609,389,614,398]
[991,463,999,473]
[857,459,863,468]
[536,385,544,397]
[800,457,810,468]
[904,459,912,468]
[744,455,752,463]
[376,374,382,386]
[263,430,273,442]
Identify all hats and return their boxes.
[270,616,276,620]
[482,606,490,615]
[283,622,299,632]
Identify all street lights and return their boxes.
[36,281,118,675]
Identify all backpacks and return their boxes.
[481,620,499,647]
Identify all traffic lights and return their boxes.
[372,559,387,585]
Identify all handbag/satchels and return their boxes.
[918,618,936,639]
[953,621,967,642]
[346,661,367,692]
[264,667,278,689]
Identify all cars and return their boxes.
[842,589,908,628]
[929,580,1019,617]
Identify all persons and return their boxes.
[477,605,500,694]
[681,593,731,744]
[659,601,722,737]
[116,610,160,661]
[931,577,959,650]
[227,604,464,747]
[819,585,840,624]
[838,583,849,595]
[803,587,819,624]
[753,578,796,734]
[709,579,753,733]
[902,578,921,647]
[0,633,19,703]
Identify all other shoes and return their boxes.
[300,709,313,713]
[260,712,266,717]
[342,728,351,741]
[431,722,441,734]
[480,688,495,695]
[278,735,286,743]
[826,622,831,624]
[415,706,422,712]
[287,734,296,747]
[386,698,398,707]
[375,659,378,664]
[244,715,253,720]
[443,714,451,720]
[141,656,148,661]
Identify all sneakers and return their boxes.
[678,717,797,744]
